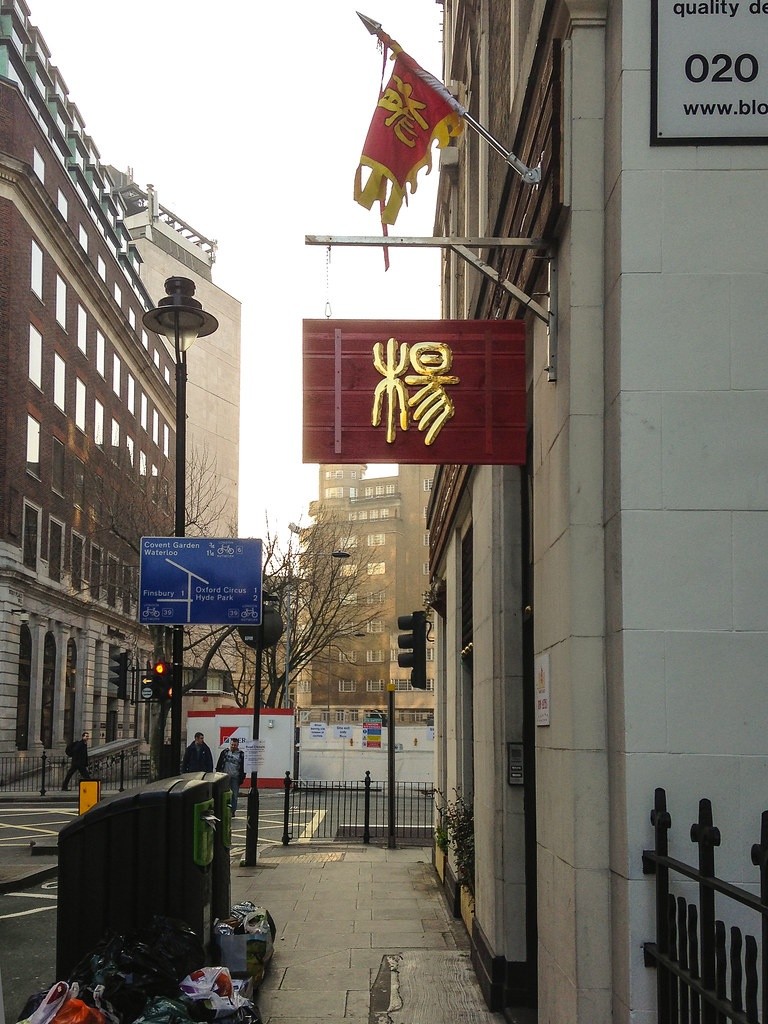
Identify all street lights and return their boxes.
[284,550,351,711]
[140,275,220,777]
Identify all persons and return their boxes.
[181,732,213,773]
[216,738,244,817]
[62,732,93,791]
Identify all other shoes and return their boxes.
[231,812,235,817]
[62,788,71,791]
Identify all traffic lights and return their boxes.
[161,683,176,699]
[397,611,427,688]
[154,661,168,676]
[106,650,129,701]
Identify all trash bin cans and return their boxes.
[55,771,232,1022]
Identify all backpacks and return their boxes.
[66,741,77,756]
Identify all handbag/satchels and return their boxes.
[215,911,271,989]
